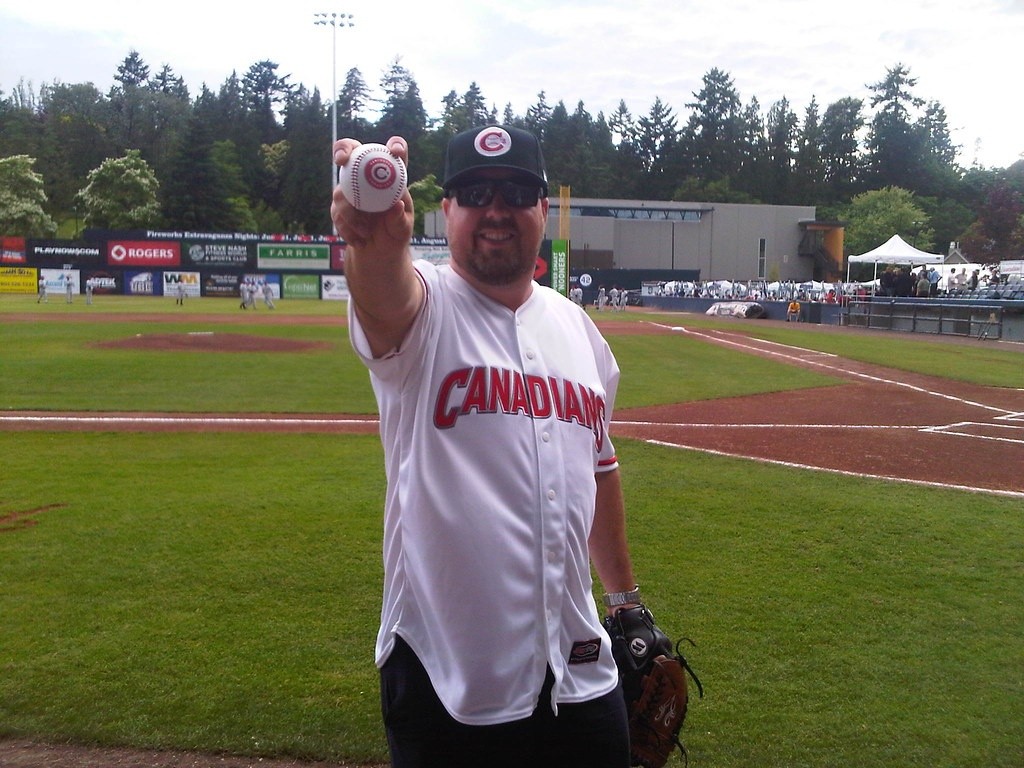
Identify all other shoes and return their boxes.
[797,319,800,322]
[786,319,790,322]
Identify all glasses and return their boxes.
[448,175,544,208]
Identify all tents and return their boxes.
[847,234,945,290]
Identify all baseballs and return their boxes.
[338,142,409,214]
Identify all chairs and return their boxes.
[933,272,1024,301]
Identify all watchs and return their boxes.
[603,584,641,607]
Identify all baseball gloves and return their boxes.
[602,608,693,768]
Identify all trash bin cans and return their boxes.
[838,312,849,326]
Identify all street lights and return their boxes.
[313,11,356,240]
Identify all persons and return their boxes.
[240,280,277,311]
[827,289,836,303]
[64,276,75,304]
[176,276,186,305]
[86,276,93,305]
[785,298,800,322]
[880,264,1007,297]
[37,275,48,303]
[331,125,689,768]
[570,284,628,312]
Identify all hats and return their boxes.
[442,125,548,188]
